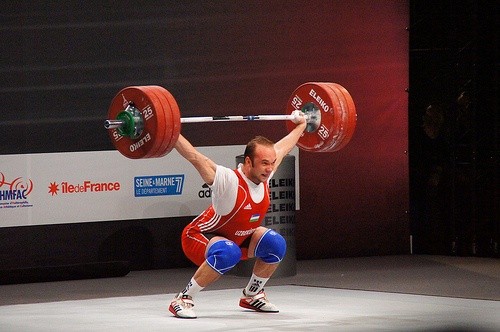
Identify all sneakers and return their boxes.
[169,291,197,318]
[239,289,279,312]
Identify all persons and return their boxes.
[169,110,307,318]
[415,87,495,255]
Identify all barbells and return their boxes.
[104,82,358,161]
